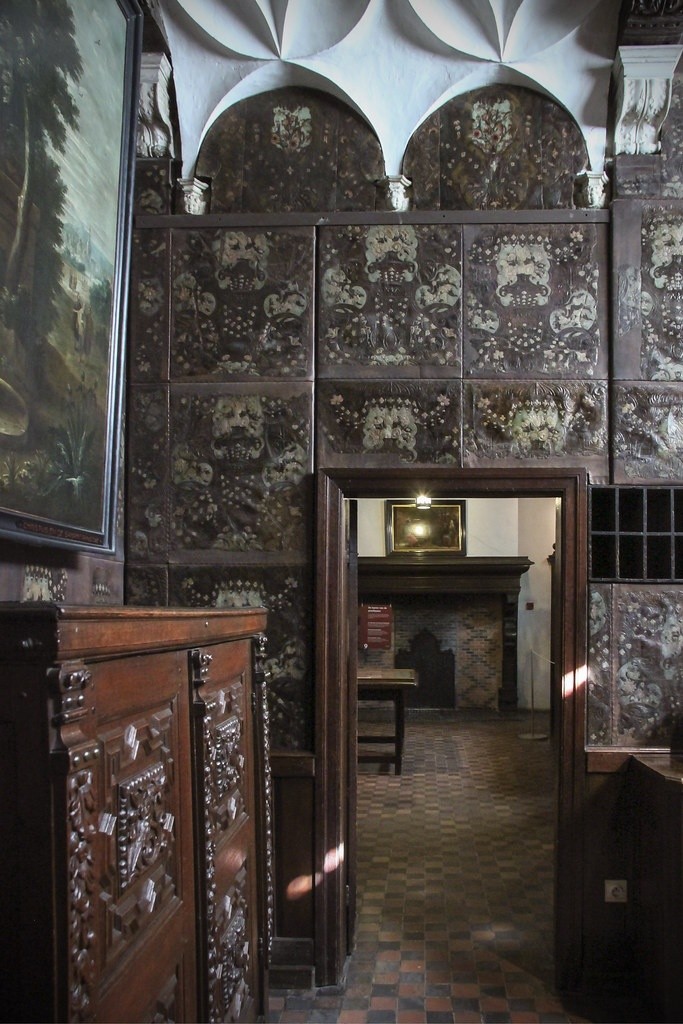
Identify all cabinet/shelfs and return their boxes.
[1,601,276,1022]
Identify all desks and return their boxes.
[358,667,418,776]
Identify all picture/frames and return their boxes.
[1,0,144,556]
[386,500,467,557]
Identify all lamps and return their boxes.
[415,495,432,509]
